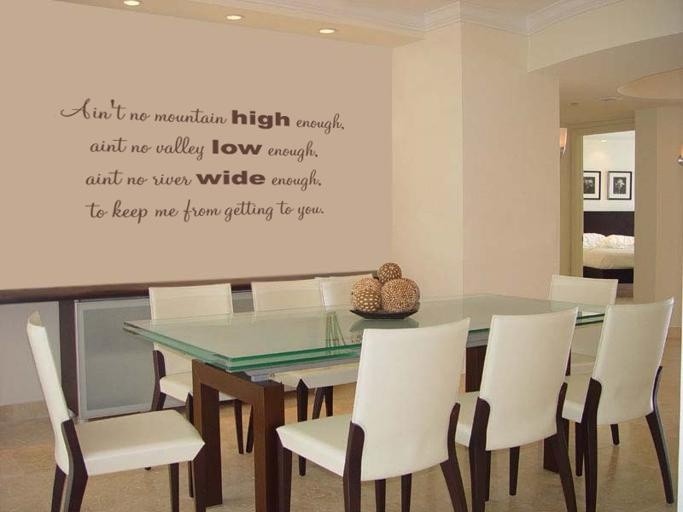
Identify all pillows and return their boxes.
[597,235,634,249]
[583,233,606,248]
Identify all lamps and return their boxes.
[560,128,567,156]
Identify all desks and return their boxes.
[120,291,613,512]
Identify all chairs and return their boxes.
[313,275,383,418]
[276,317,470,512]
[142,284,243,470]
[245,278,325,477]
[505,295,674,505]
[26,310,207,512]
[549,273,621,477]
[447,304,580,511]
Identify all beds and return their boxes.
[584,211,634,283]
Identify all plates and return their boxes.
[348,317,419,332]
[348,307,420,319]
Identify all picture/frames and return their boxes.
[607,171,632,200]
[584,171,601,200]
